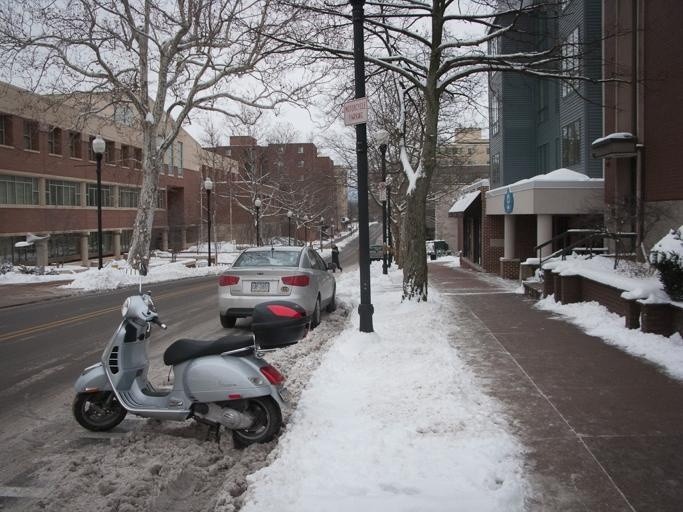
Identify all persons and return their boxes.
[330,243,342,272]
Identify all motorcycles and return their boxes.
[71,263,309,445]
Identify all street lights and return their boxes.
[204,177,213,267]
[92,134,106,270]
[254,197,262,247]
[287,210,293,246]
[303,215,324,253]
[376,130,393,275]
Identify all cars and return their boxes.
[216,238,337,332]
[426,239,452,256]
[369,245,384,264]
